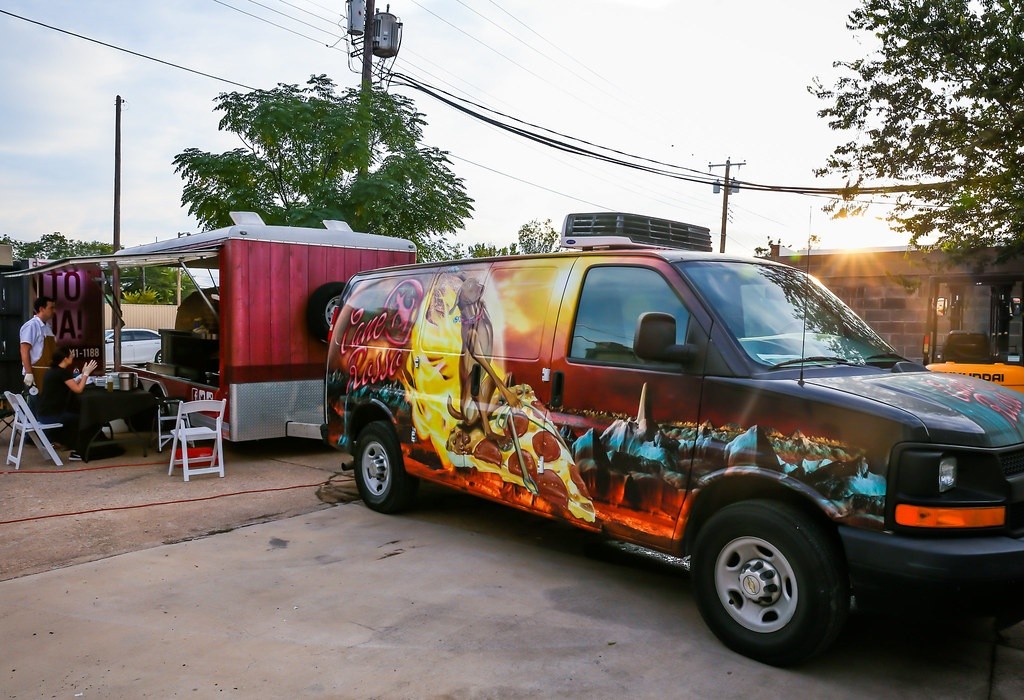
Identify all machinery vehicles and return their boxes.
[917,272,1024,397]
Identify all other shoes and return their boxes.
[24,437,35,445]
[43,444,54,448]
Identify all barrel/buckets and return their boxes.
[118,373,134,391]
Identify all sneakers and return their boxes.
[69,451,82,460]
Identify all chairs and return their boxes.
[168,399,227,482]
[4,391,63,470]
[941,330,992,363]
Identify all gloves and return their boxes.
[23,374,36,386]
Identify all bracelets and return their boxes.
[83,373,88,377]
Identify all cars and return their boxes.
[105,329,161,367]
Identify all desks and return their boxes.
[69,383,157,463]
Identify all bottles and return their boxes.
[73,365,80,378]
[107,375,113,392]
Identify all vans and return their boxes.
[316,211,1024,668]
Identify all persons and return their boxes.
[18,296,98,462]
